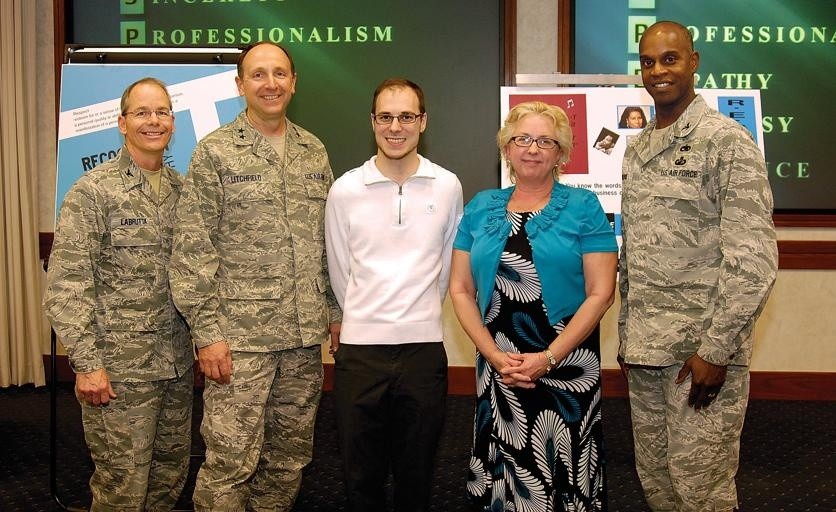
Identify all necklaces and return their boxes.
[512,193,552,211]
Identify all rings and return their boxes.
[707,393,714,398]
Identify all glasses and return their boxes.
[372,112,424,124]
[509,135,560,150]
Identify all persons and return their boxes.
[596,135,614,154]
[324,77,465,512]
[169,42,335,511]
[618,106,647,128]
[617,20,779,512]
[449,101,612,511]
[42,76,187,512]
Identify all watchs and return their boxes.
[544,350,556,374]
